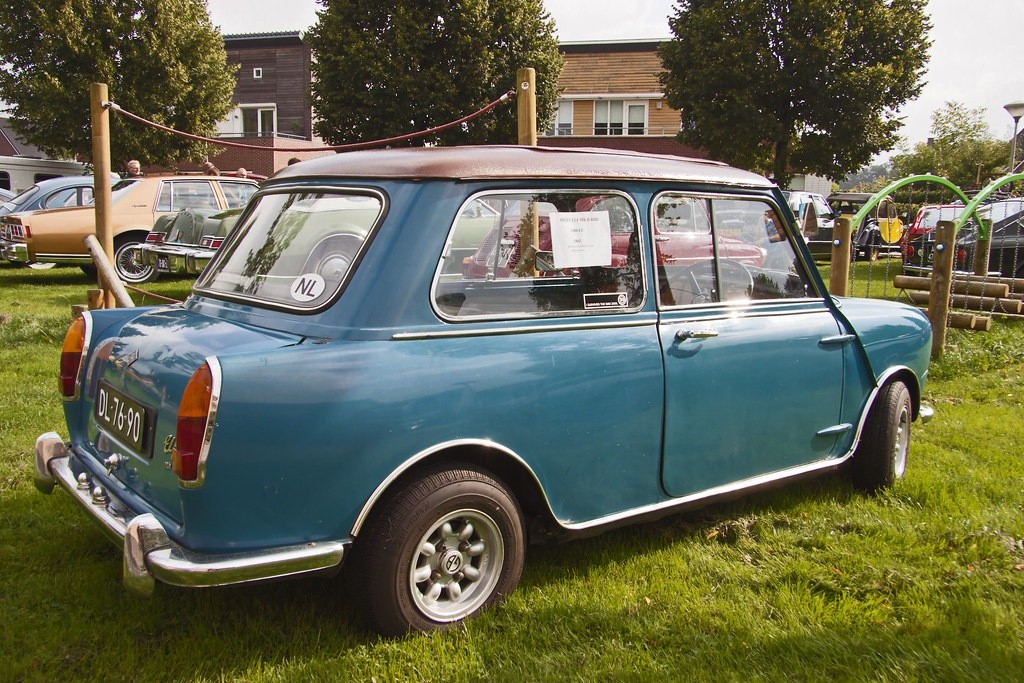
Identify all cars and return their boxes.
[899,205,985,264]
[463,192,770,306]
[713,190,853,271]
[0,177,122,271]
[907,197,1024,281]
[825,191,905,261]
[143,191,504,304]
[0,175,261,286]
[25,143,935,643]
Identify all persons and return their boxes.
[227,166,253,209]
[190,157,223,206]
[125,159,144,181]
[288,158,302,169]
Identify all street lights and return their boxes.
[1003,100,1024,175]
